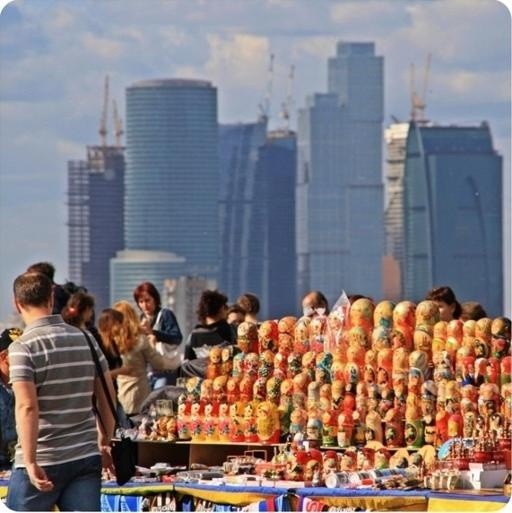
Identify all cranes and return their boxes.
[407,53,433,124]
[99,66,124,149]
[260,49,295,137]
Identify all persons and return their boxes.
[4,271,118,511]
[1,264,491,472]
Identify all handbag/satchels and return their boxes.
[110,436,137,486]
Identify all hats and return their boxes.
[0,327,24,351]
